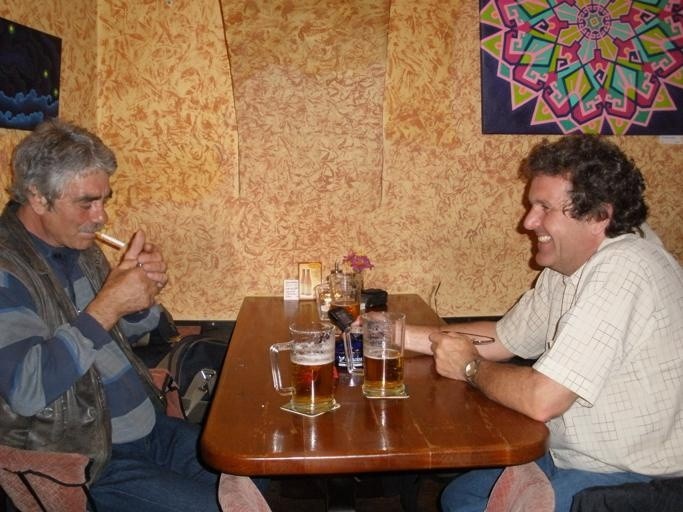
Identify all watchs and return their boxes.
[464,358,484,385]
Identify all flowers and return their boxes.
[339,246,378,276]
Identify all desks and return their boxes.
[194,291,552,512]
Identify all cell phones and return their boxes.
[328,308,362,339]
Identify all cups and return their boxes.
[268,318,335,411]
[270,412,335,453]
[342,310,407,397]
[363,400,404,449]
[314,268,390,319]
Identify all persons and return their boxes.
[1,118,271,511]
[351,132,683,511]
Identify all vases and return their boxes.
[344,270,365,297]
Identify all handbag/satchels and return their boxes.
[146,334,227,423]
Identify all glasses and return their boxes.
[434,281,494,345]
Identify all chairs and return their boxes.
[1,321,203,512]
[219,461,556,512]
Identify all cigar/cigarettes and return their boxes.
[94,231,127,251]
